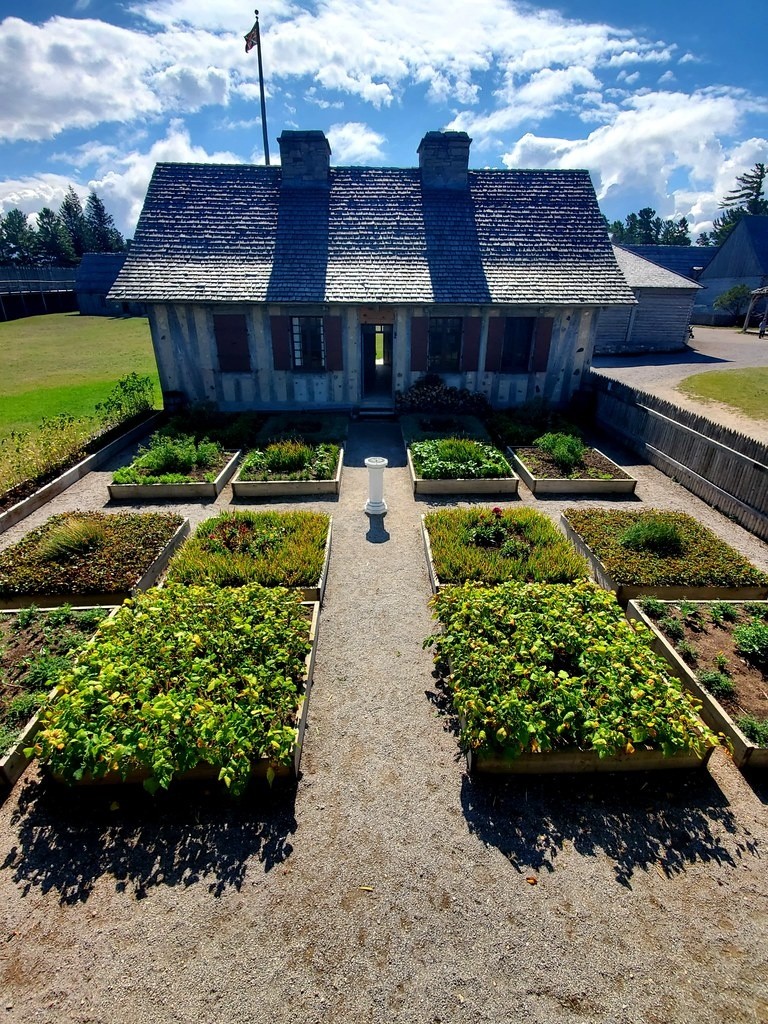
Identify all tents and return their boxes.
[743,286,768,332]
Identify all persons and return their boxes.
[759,318,767,339]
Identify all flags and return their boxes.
[244,22,260,53]
